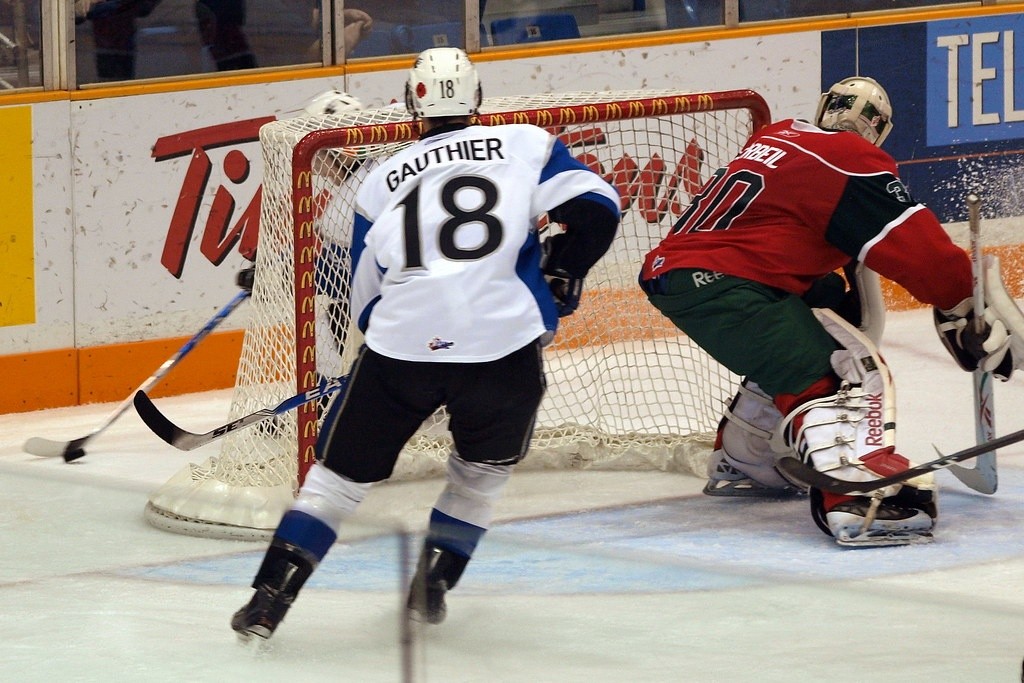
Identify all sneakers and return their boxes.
[407,545,470,625]
[231,538,320,649]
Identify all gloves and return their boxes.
[933,254,1024,383]
[537,268,583,317]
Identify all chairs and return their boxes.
[349,14,580,56]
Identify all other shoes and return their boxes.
[702,419,809,499]
[808,454,938,546]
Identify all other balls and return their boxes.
[63,449,85,463]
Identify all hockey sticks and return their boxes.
[933,190,999,497]
[774,430,1023,501]
[21,284,251,458]
[134,376,347,454]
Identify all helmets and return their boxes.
[815,76,893,147]
[405,48,482,117]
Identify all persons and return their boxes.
[640,77,1013,547]
[88,0,257,82]
[233,47,621,638]
[254,1,370,82]
